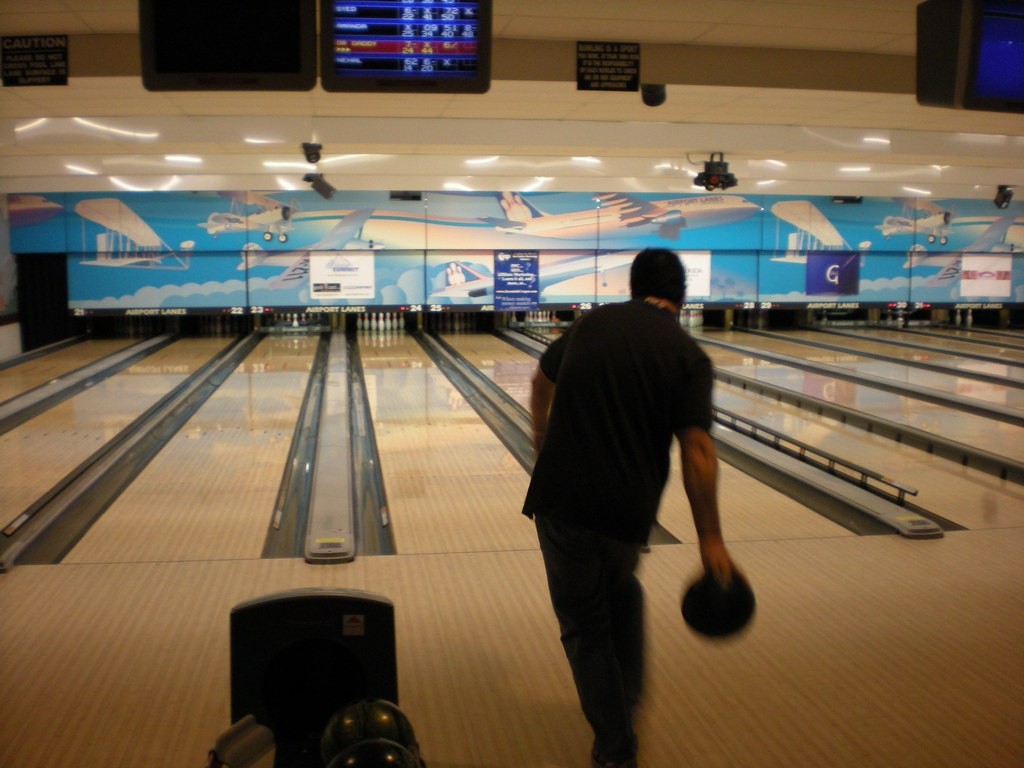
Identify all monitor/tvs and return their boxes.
[914,1,1023,114]
[140,1,317,92]
[319,0,491,94]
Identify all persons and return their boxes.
[521,246,733,767]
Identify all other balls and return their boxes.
[682,573,756,636]
[321,698,420,767]
